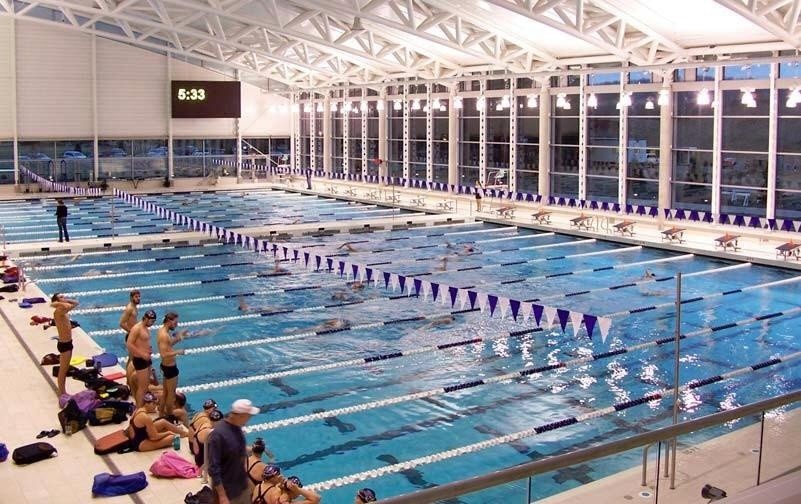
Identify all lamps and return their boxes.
[303,66,755,111]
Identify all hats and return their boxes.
[232,399,260,415]
[143,394,154,402]
[203,399,216,410]
[210,411,222,421]
[285,476,302,490]
[263,465,279,480]
[253,440,265,452]
[357,489,376,502]
[145,311,156,319]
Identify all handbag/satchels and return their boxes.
[87,376,130,399]
[58,399,130,436]
[13,442,54,465]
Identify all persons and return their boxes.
[240,264,456,330]
[187,398,378,503]
[305,166,312,190]
[120,290,188,452]
[52,293,80,396]
[474,181,484,212]
[337,243,356,252]
[54,200,69,242]
[463,243,474,254]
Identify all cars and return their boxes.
[175,146,209,162]
[64,151,86,159]
[18,152,48,160]
[723,158,735,169]
[106,148,126,157]
[147,149,167,156]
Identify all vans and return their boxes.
[646,154,657,166]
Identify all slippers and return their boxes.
[37,429,60,438]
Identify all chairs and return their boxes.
[321,182,801,264]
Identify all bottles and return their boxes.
[97,361,102,372]
[173,434,180,450]
[64,422,72,437]
